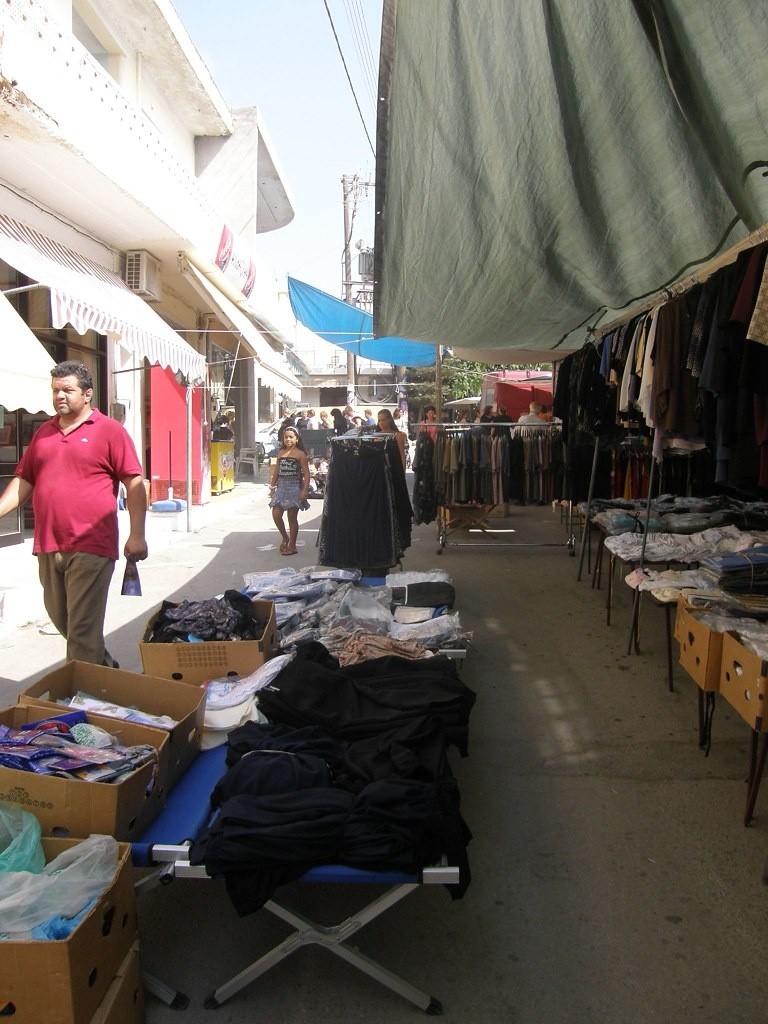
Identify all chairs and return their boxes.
[234,442,263,481]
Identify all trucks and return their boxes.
[257,405,384,458]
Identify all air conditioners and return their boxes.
[125,249,162,302]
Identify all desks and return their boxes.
[574,498,768,694]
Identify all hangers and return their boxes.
[616,442,654,460]
[639,268,699,312]
[331,423,396,444]
[572,318,621,351]
[413,422,564,439]
[749,224,768,245]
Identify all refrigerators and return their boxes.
[210,440,235,496]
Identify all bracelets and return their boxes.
[269,486,277,489]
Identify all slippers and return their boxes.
[280,539,298,555]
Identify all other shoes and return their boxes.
[113,660,119,669]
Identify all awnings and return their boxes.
[0,291,58,416]
[0,214,207,383]
[177,253,302,403]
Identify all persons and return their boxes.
[0,361,150,670]
[267,426,310,555]
[278,405,375,447]
[392,408,409,436]
[213,410,235,440]
[419,401,554,445]
[376,409,406,473]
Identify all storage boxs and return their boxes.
[140,597,275,683]
[19,659,207,781]
[0,836,137,1024]
[89,941,145,1024]
[673,592,768,734]
[0,704,170,844]
[438,503,510,534]
